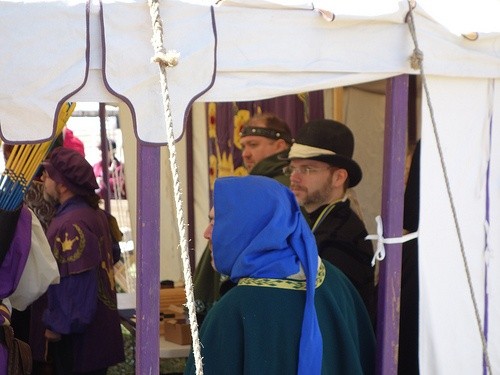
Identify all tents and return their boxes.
[0,0,499,375]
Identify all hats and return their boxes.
[97,139,117,151]
[40,147,100,209]
[276,119,363,188]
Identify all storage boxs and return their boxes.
[159,336,190,375]
[164,320,191,344]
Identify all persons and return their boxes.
[191,115,292,322]
[185,175,380,375]
[90,138,127,200]
[33,146,125,375]
[4,128,83,231]
[0,190,60,375]
[288,118,374,298]
